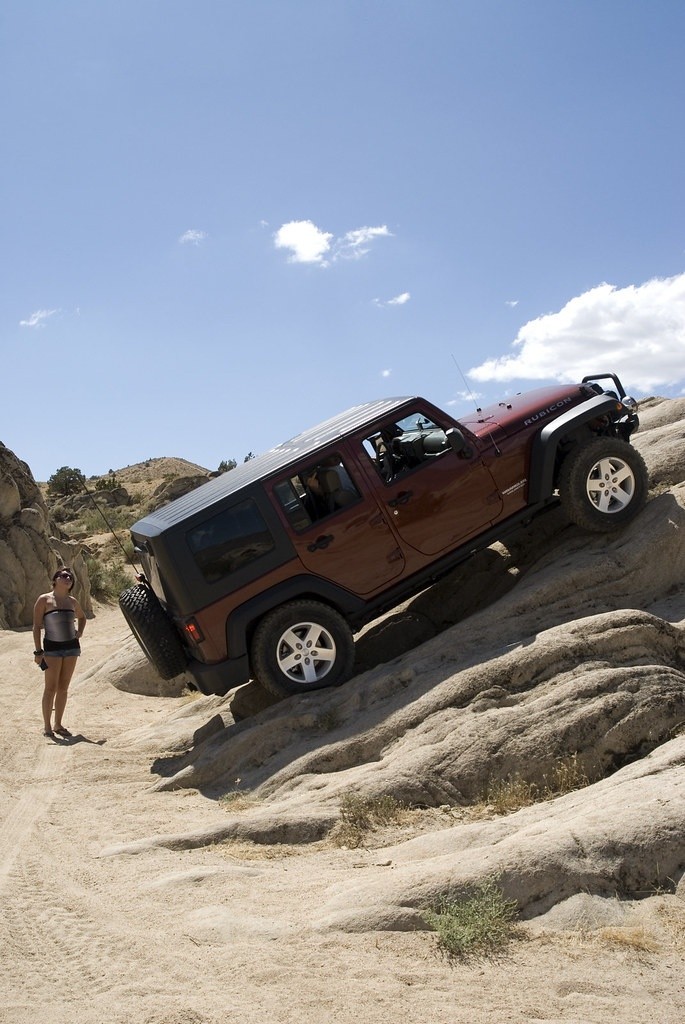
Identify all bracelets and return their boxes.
[34,649,43,655]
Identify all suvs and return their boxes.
[119,373,649,700]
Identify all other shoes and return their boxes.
[43,733,57,745]
[54,729,72,739]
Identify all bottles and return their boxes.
[33,650,48,671]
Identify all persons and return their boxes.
[320,455,390,490]
[33,568,86,745]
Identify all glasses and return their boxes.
[58,574,73,581]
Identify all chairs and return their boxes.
[306,470,355,520]
